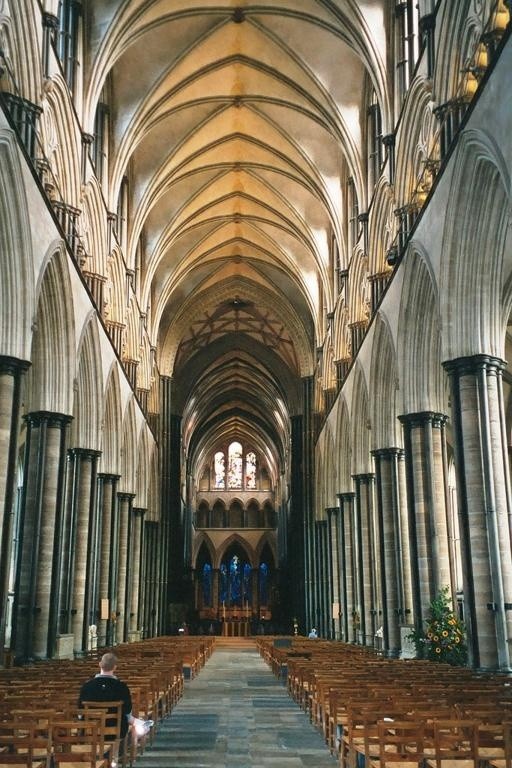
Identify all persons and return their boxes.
[76,653,132,766]
[309,628,317,639]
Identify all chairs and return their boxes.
[0,634,217,767]
[258,634,512,767]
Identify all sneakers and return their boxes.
[137,720,153,735]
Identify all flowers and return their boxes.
[404,585,467,662]
